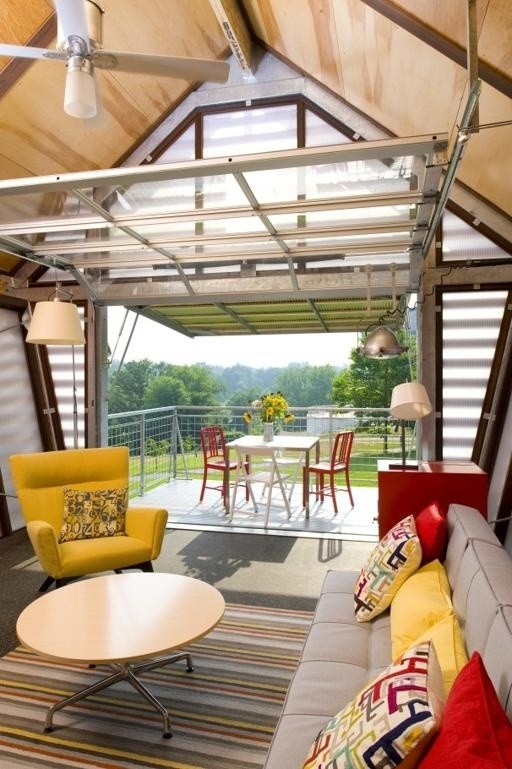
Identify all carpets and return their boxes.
[1,599,314,768]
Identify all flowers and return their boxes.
[243,392,293,424]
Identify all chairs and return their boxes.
[7,444,168,592]
[199,424,354,519]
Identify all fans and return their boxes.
[0,1,230,120]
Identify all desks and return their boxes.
[222,434,320,534]
[375,458,489,542]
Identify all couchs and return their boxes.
[261,502,512,768]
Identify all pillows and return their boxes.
[54,487,131,545]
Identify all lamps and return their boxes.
[361,265,454,361]
[23,255,86,347]
[61,54,98,120]
[388,382,432,469]
